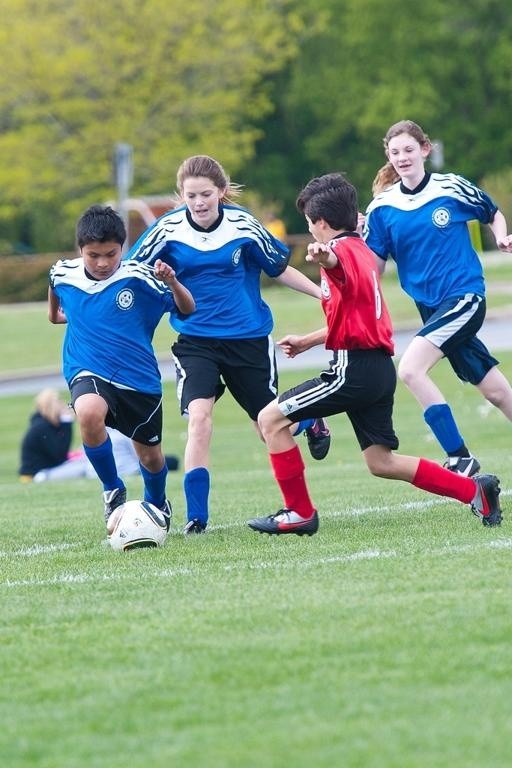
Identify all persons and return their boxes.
[355,119,511,477]
[20,390,180,479]
[48,207,196,535]
[248,173,503,535]
[118,156,329,533]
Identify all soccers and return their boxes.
[105,499,167,553]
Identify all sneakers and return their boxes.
[468,474,503,528]
[303,415,332,461]
[101,486,127,525]
[183,518,207,535]
[442,452,481,478]
[248,507,319,537]
[158,499,173,535]
